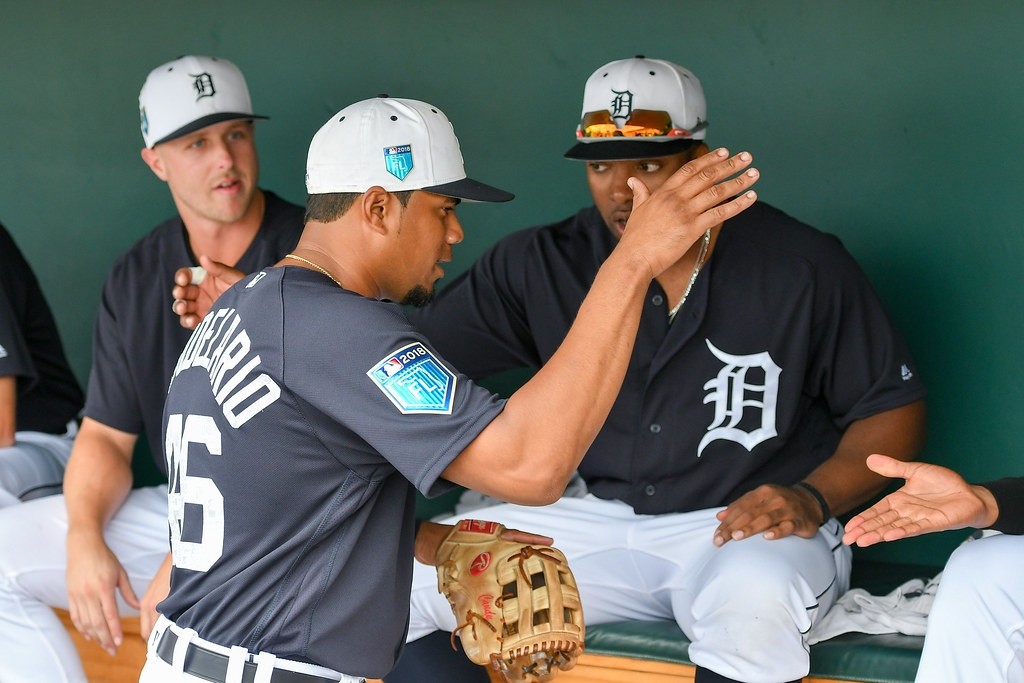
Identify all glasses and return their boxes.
[579,109,709,140]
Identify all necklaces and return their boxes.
[667,228,711,324]
[284,253,342,286]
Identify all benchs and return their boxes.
[51,557,945,683]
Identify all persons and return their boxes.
[140,92,760,683]
[0,222,88,507]
[843,454,1024,683]
[168,52,927,683]
[0,55,309,682]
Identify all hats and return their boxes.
[565,54,707,161]
[305,96,516,204]
[138,56,270,150]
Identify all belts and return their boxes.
[156,627,340,683]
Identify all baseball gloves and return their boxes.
[434,518,586,683]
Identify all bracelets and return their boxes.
[790,482,828,526]
